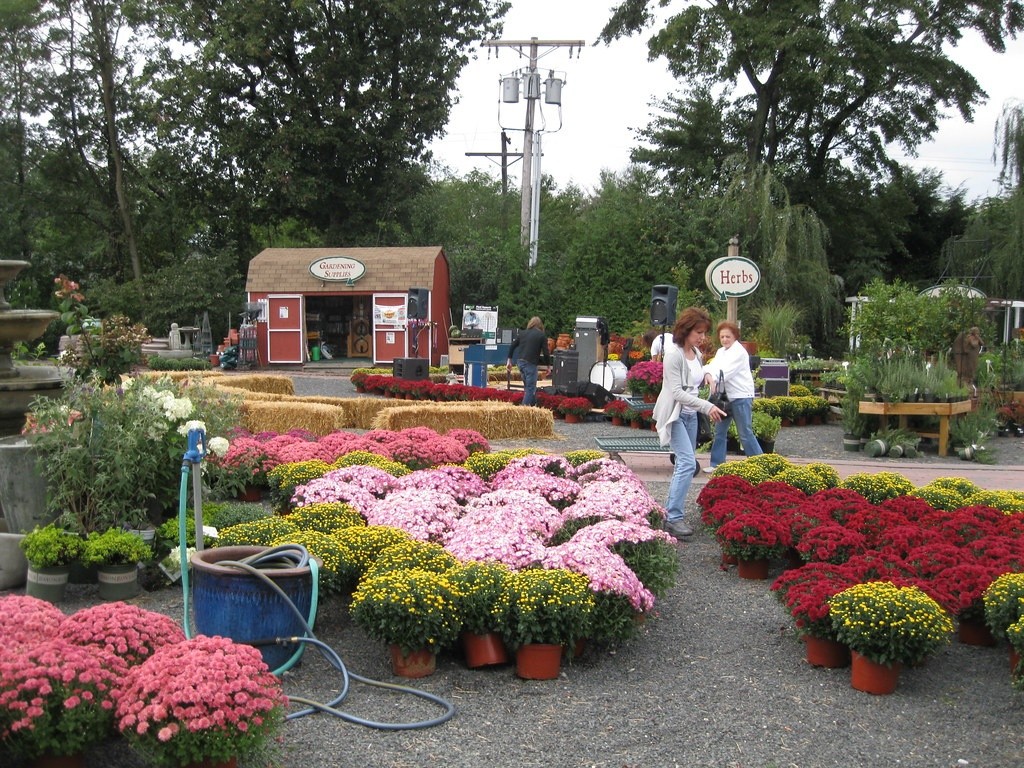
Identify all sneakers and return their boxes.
[664,518,694,541]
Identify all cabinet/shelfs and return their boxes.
[307,319,330,360]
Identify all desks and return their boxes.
[446,337,487,378]
[856,399,973,458]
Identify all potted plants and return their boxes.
[86,520,155,600]
[17,522,84,602]
[728,275,1013,461]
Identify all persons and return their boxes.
[644,331,673,361]
[506,316,551,406]
[702,321,763,473]
[651,306,727,537]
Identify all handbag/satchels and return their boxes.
[709,370,732,420]
[696,412,713,450]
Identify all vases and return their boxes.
[810,414,823,425]
[953,610,1000,648]
[357,385,365,393]
[566,411,580,424]
[210,354,220,365]
[650,422,657,431]
[404,392,413,400]
[385,639,440,678]
[795,415,807,426]
[806,416,811,425]
[420,395,429,401]
[781,417,793,427]
[631,421,641,429]
[374,388,381,394]
[1007,644,1021,673]
[849,649,900,695]
[186,749,244,768]
[611,417,622,425]
[384,389,392,398]
[395,392,402,399]
[438,397,444,401]
[721,545,737,565]
[806,629,850,668]
[464,625,511,669]
[513,642,563,681]
[736,549,770,578]
[554,411,562,418]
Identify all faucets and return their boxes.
[180,427,208,474]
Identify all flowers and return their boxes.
[0,361,1024,768]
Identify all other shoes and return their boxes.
[703,466,716,473]
